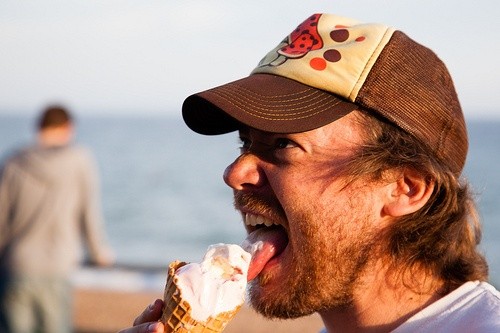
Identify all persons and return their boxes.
[117,13,500,333]
[0,106,111,333]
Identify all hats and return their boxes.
[182,13,469,181]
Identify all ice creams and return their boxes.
[156,242,252,333]
